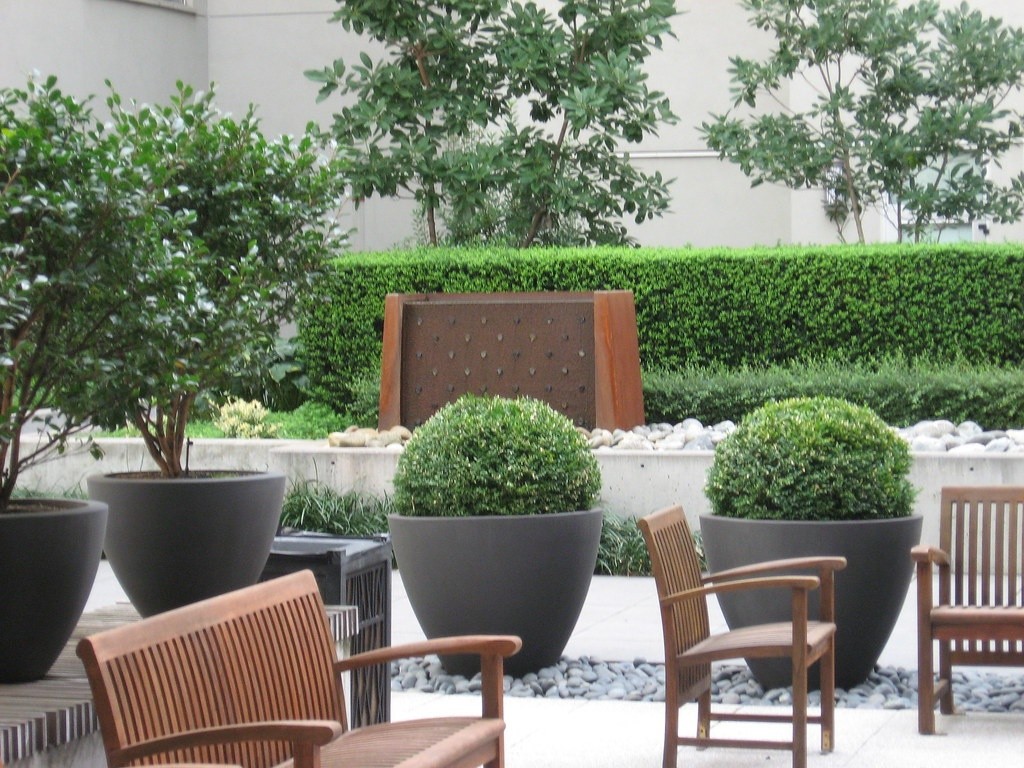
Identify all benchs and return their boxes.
[75,568,523,767]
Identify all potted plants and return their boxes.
[697,394,926,695]
[46,74,357,622]
[386,393,603,677]
[1,74,225,684]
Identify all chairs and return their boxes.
[909,482,1023,734]
[636,505,847,768]
